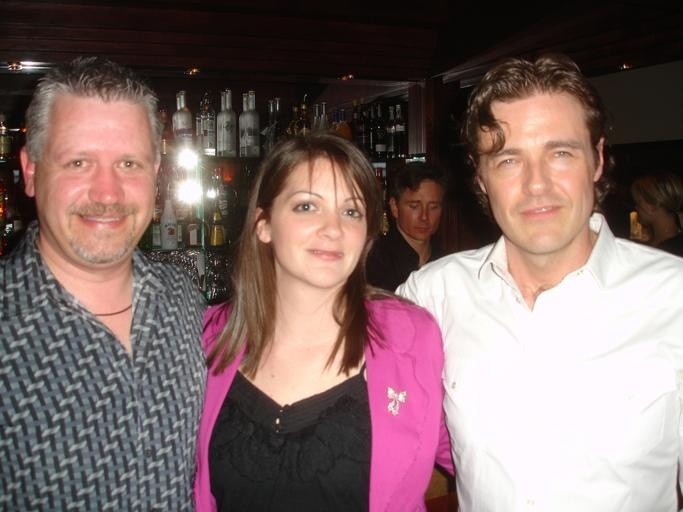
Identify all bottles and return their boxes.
[381,187,389,238]
[207,198,225,246]
[159,197,177,251]
[182,199,203,248]
[0,117,31,260]
[175,88,405,159]
[157,105,171,154]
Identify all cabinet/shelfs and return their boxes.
[1,0,683,302]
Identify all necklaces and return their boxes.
[93,304,132,318]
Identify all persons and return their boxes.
[194,130,455,507]
[368,161,457,294]
[626,172,681,255]
[393,58,683,508]
[0,54,212,508]
